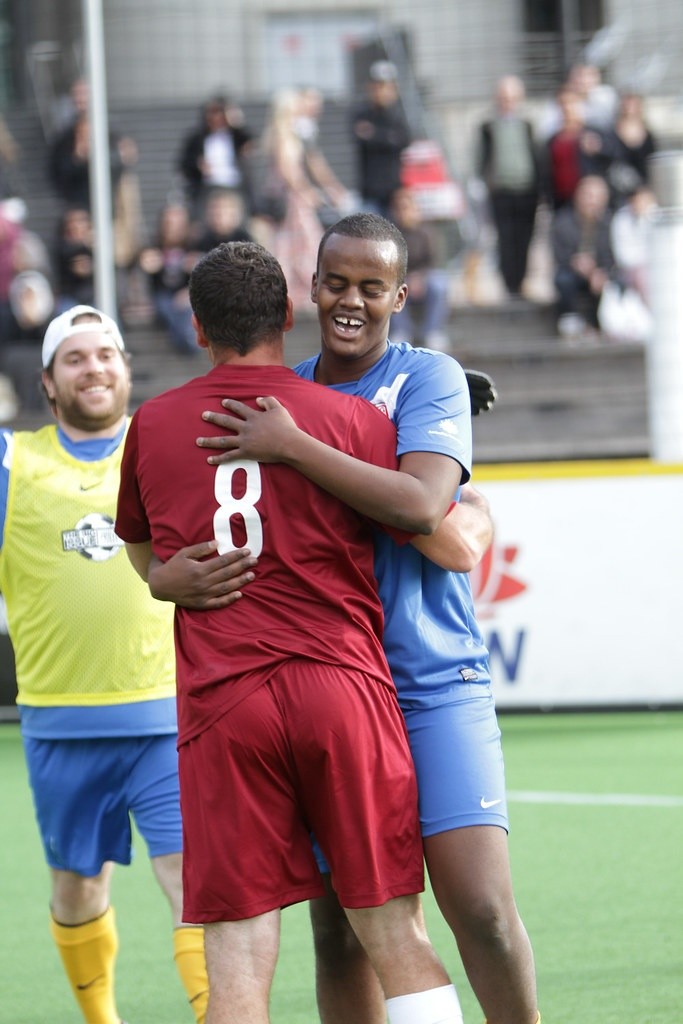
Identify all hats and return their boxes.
[41,305,125,371]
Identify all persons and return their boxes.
[0,305,211,1024]
[115,241,496,1024]
[0,64,659,417]
[148,214,540,1024]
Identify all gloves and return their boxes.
[463,369,495,415]
